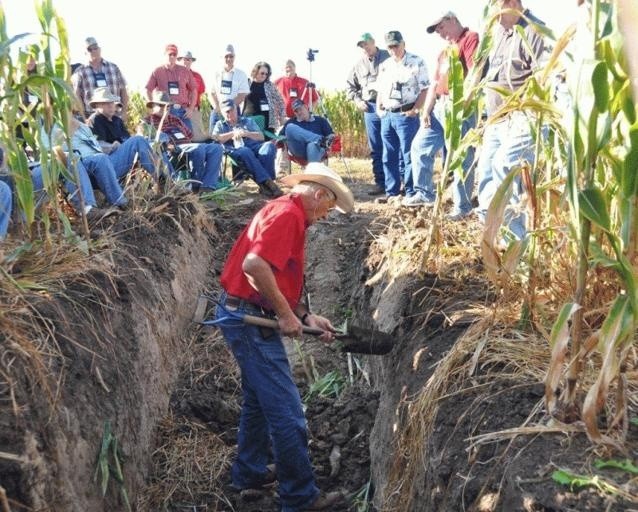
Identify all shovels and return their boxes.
[190,295,394,354]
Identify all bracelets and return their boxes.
[300,311,314,324]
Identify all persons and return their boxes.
[345,0,562,252]
[213,161,353,512]
[0,38,342,243]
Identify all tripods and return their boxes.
[276,62,356,188]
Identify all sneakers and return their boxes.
[260,179,283,198]
[307,491,339,511]
[87,208,105,220]
[262,463,277,481]
[447,208,472,220]
[405,194,434,207]
[368,185,384,195]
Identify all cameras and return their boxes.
[307,49,314,62]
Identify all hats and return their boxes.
[426,11,455,34]
[283,163,354,214]
[165,45,178,57]
[356,33,373,44]
[146,91,171,106]
[385,31,401,46]
[219,99,233,113]
[90,87,115,108]
[179,52,197,61]
[82,37,97,50]
[291,99,304,112]
[222,45,235,56]
